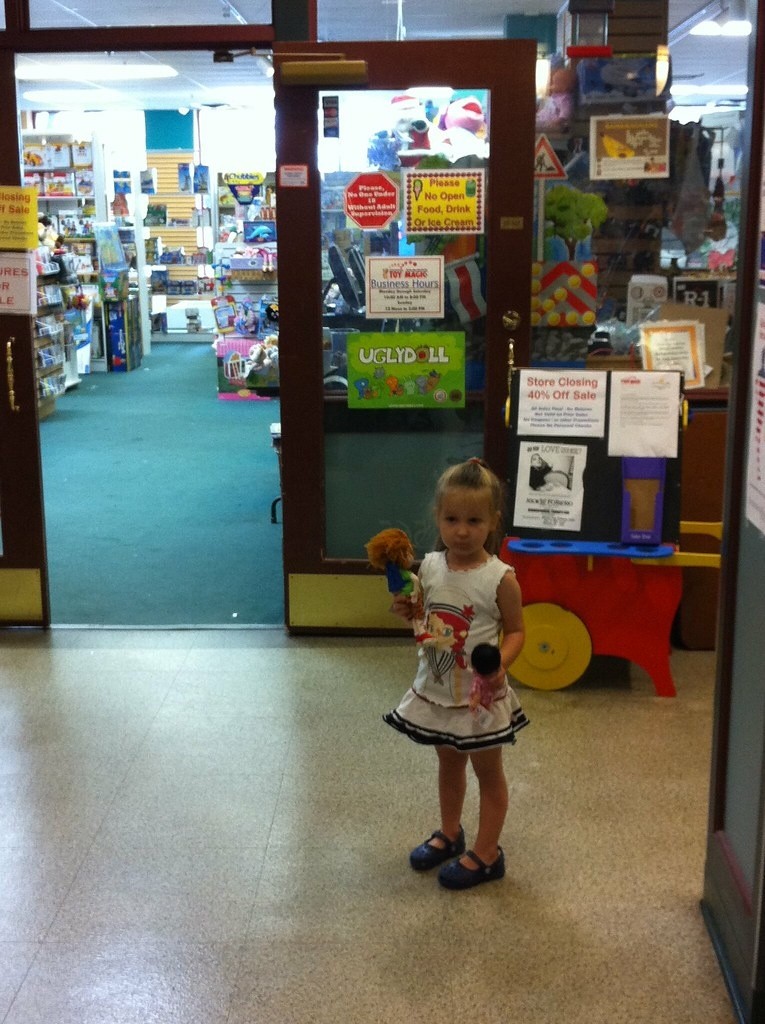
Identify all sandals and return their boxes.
[410,824,465,871]
[438,846,505,888]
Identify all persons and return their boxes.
[382,457,532,890]
[529,452,554,491]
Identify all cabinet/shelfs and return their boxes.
[20,127,153,422]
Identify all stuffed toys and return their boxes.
[467,641,508,709]
[250,335,278,369]
[364,527,435,646]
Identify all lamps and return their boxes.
[255,56,276,78]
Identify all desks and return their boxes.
[498,535,682,698]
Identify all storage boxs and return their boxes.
[626,273,670,329]
